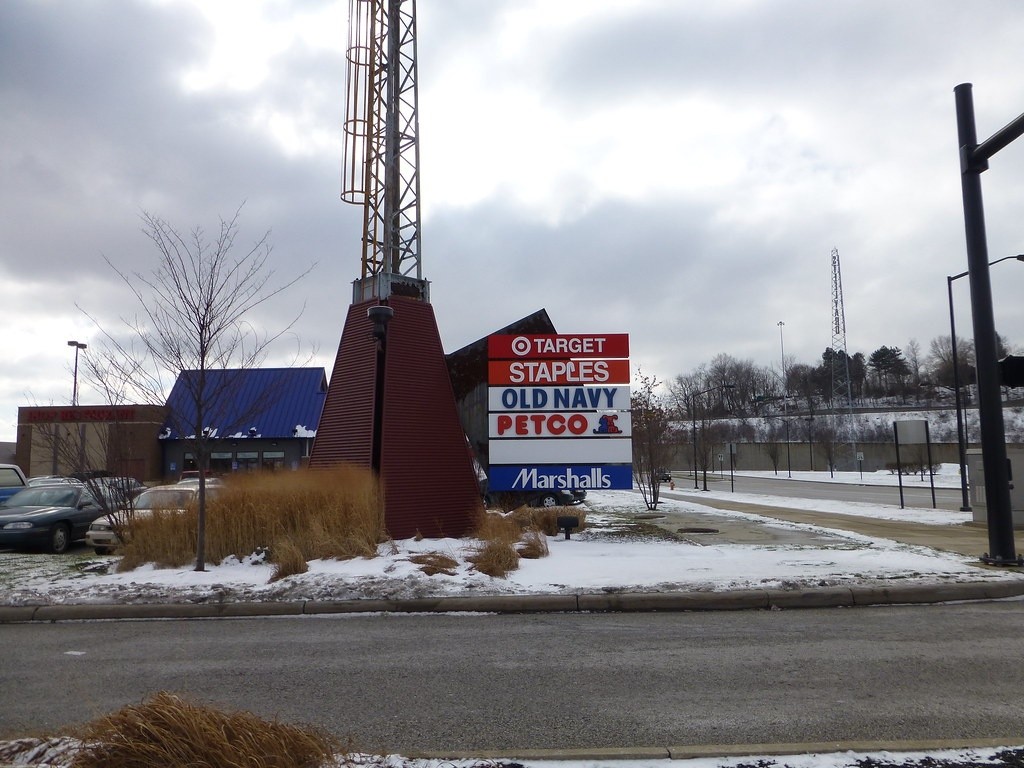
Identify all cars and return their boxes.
[86,477,223,555]
[85,477,147,501]
[656,468,671,482]
[0,476,117,553]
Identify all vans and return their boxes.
[1,464,30,504]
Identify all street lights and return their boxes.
[947,255,1024,512]
[69,341,87,405]
[692,385,735,489]
